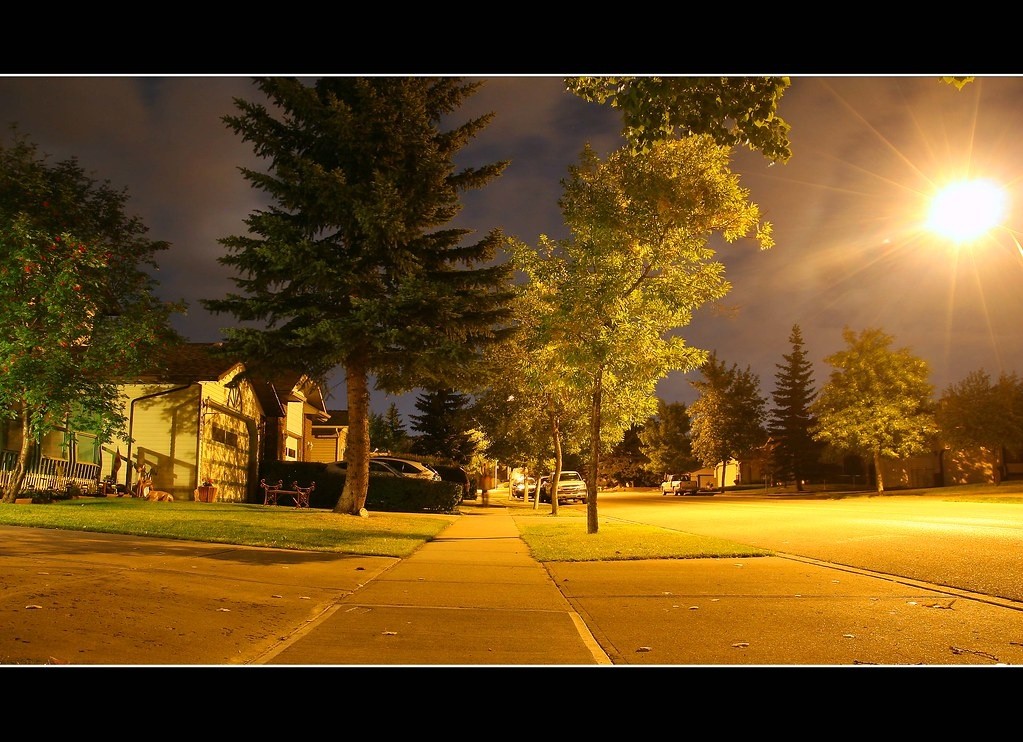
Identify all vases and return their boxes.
[198,486,218,503]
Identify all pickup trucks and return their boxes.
[660,474,697,496]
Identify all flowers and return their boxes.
[201,477,213,486]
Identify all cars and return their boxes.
[334,458,471,505]
[544,471,588,505]
[511,476,548,502]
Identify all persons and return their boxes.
[481,474,491,508]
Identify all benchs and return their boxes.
[260,479,316,509]
